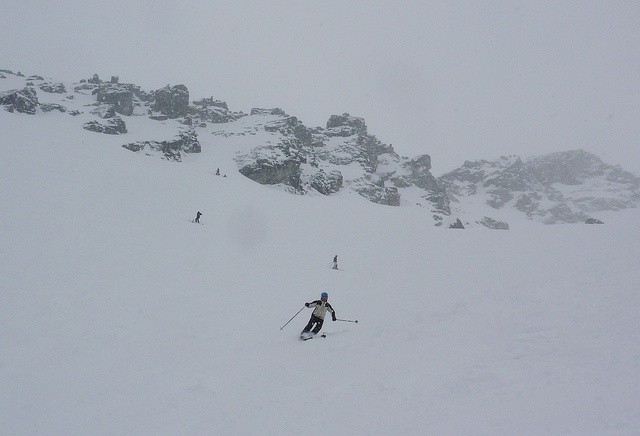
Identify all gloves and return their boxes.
[332,317,337,321]
[305,302,309,306]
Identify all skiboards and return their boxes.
[300,332,326,341]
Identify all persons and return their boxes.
[196,212,202,222]
[333,255,338,269]
[302,292,336,335]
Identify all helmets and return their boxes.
[322,292,328,297]
